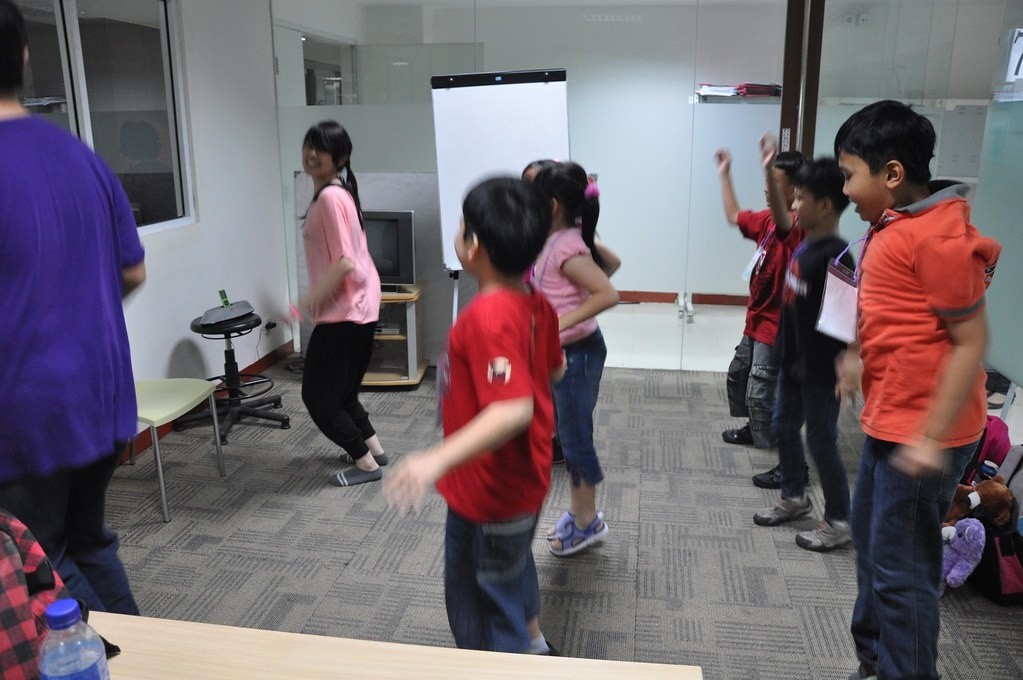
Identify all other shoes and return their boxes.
[537,640,561,657]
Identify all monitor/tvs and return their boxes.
[360,208,415,293]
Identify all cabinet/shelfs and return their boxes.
[360,280,430,391]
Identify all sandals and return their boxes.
[546,512,609,556]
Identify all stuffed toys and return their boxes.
[939,475,1014,601]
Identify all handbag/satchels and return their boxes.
[0,507,122,680]
[995,535,1023,596]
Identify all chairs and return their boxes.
[129,378,225,522]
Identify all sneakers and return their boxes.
[721,421,754,445]
[796,515,855,553]
[753,488,813,527]
[752,461,810,489]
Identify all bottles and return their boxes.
[37,597,110,679]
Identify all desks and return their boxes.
[84,611,704,680]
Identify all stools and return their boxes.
[172,316,290,446]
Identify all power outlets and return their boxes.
[264,319,278,332]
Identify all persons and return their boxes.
[0,0,147,615]
[830,100,1002,680]
[296,121,391,486]
[518,160,621,555]
[712,133,857,552]
[386,179,567,658]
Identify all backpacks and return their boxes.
[960,414,1012,489]
